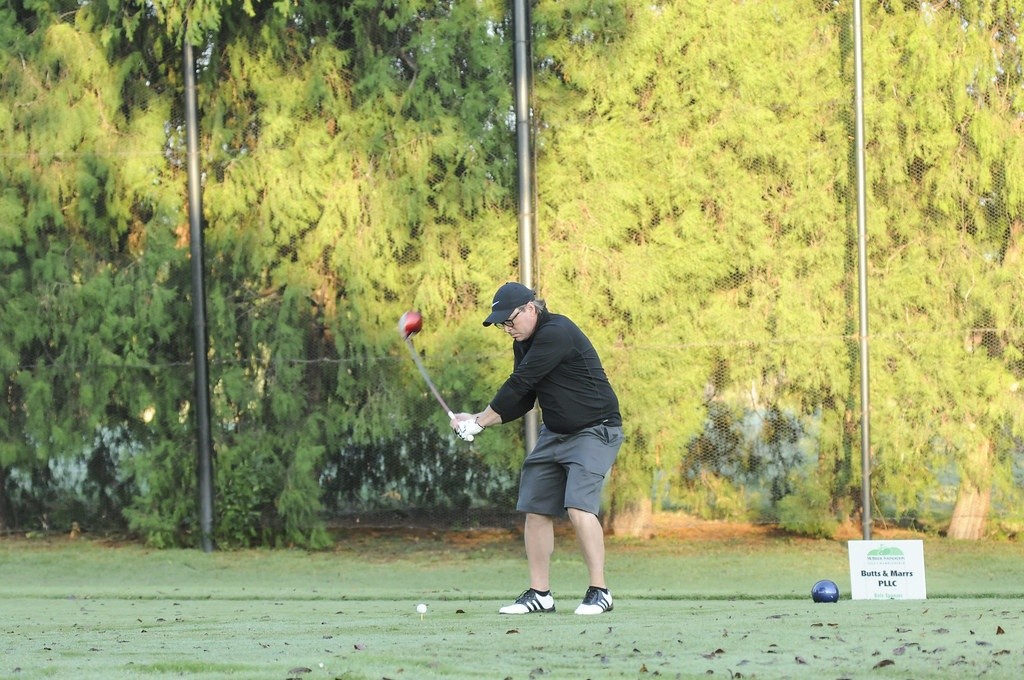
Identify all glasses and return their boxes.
[495,307,525,329]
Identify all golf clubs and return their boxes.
[397,309,476,443]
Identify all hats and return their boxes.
[483,282,534,327]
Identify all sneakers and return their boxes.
[574,587,614,614]
[499,589,555,615]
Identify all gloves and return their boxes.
[454,417,485,440]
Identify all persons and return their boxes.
[451,281,625,616]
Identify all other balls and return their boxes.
[811,579,839,603]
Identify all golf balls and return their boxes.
[417,604,427,613]
